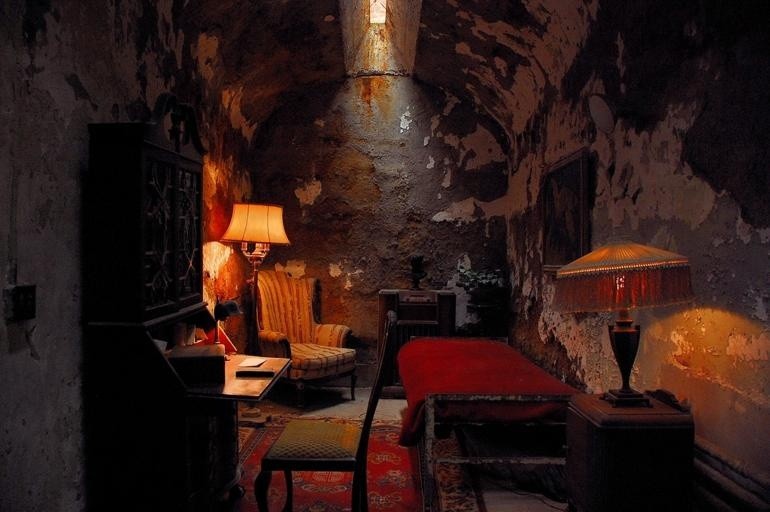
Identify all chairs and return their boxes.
[253,269,358,411]
[249,318,400,512]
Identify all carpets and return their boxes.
[212,417,426,511]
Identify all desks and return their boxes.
[82,348,292,512]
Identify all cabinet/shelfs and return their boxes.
[564,392,695,512]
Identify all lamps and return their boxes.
[553,240,697,410]
[222,204,292,428]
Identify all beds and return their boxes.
[400,335,582,511]
